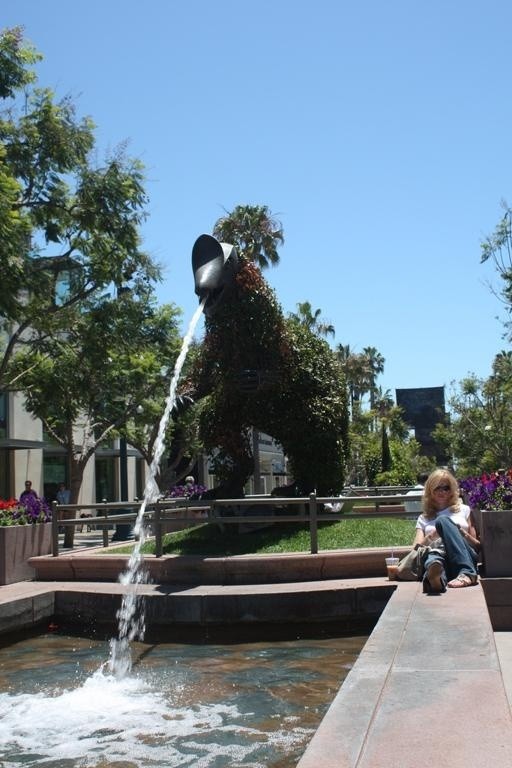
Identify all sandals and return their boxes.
[427,560,443,593]
[447,573,478,588]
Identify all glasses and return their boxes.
[434,484,450,492]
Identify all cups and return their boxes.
[386,557,400,578]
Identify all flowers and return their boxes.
[0,492,53,526]
[162,483,208,500]
[460,470,511,511]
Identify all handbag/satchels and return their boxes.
[394,543,429,582]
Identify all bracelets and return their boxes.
[426,535,433,543]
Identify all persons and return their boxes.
[184,476,196,488]
[413,468,481,593]
[57,483,72,504]
[17,481,38,502]
